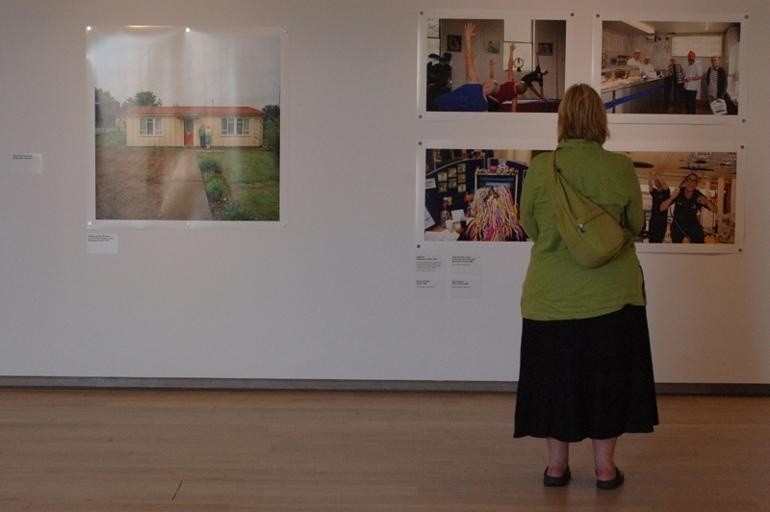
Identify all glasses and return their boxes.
[686,177,697,184]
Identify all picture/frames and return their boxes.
[85,25,290,230]
[608,144,746,254]
[417,7,577,122]
[591,12,751,124]
[415,138,556,251]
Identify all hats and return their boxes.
[688,51,695,58]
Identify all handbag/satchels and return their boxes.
[710,99,727,115]
[547,148,629,269]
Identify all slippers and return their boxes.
[595,465,624,490]
[543,465,570,487]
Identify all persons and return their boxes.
[204,125,213,147]
[684,51,703,112]
[489,59,500,82]
[519,49,549,104]
[642,56,655,73]
[648,167,670,244]
[660,174,716,244]
[705,56,728,115]
[427,22,500,113]
[627,49,643,70]
[667,57,686,107]
[513,83,660,488]
[484,40,527,112]
[199,122,205,151]
